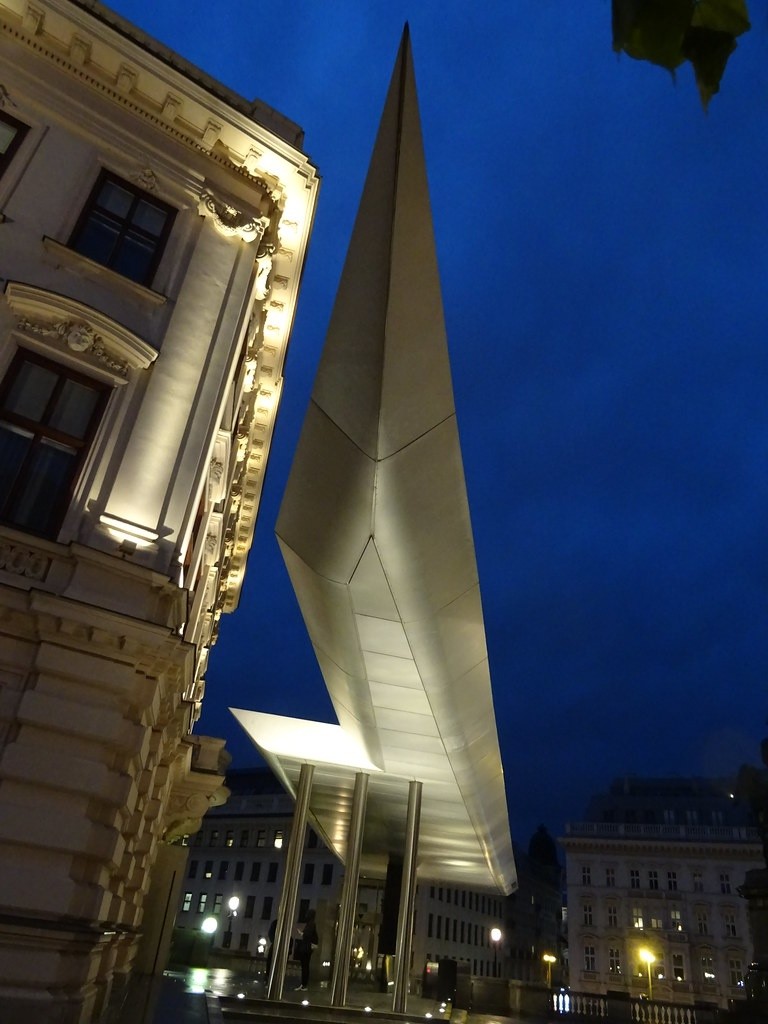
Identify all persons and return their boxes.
[264,915,289,990]
[295,909,319,996]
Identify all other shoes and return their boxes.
[293,984,303,990]
[300,988,308,991]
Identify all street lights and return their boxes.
[490,927,502,978]
[542,954,558,993]
[639,948,656,1001]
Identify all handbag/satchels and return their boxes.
[310,942,320,951]
